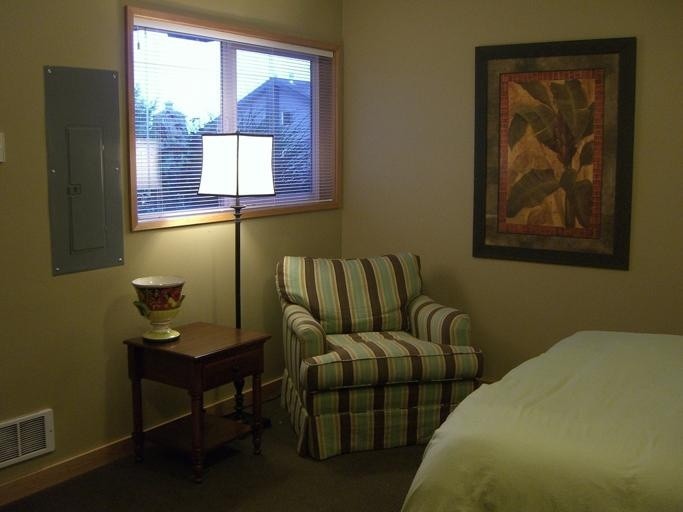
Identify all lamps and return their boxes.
[195,133,277,440]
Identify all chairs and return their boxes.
[275,253,484,460]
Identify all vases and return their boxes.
[130,275,186,343]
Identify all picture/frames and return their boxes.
[471,36,634,271]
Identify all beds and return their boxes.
[398,330,682,512]
